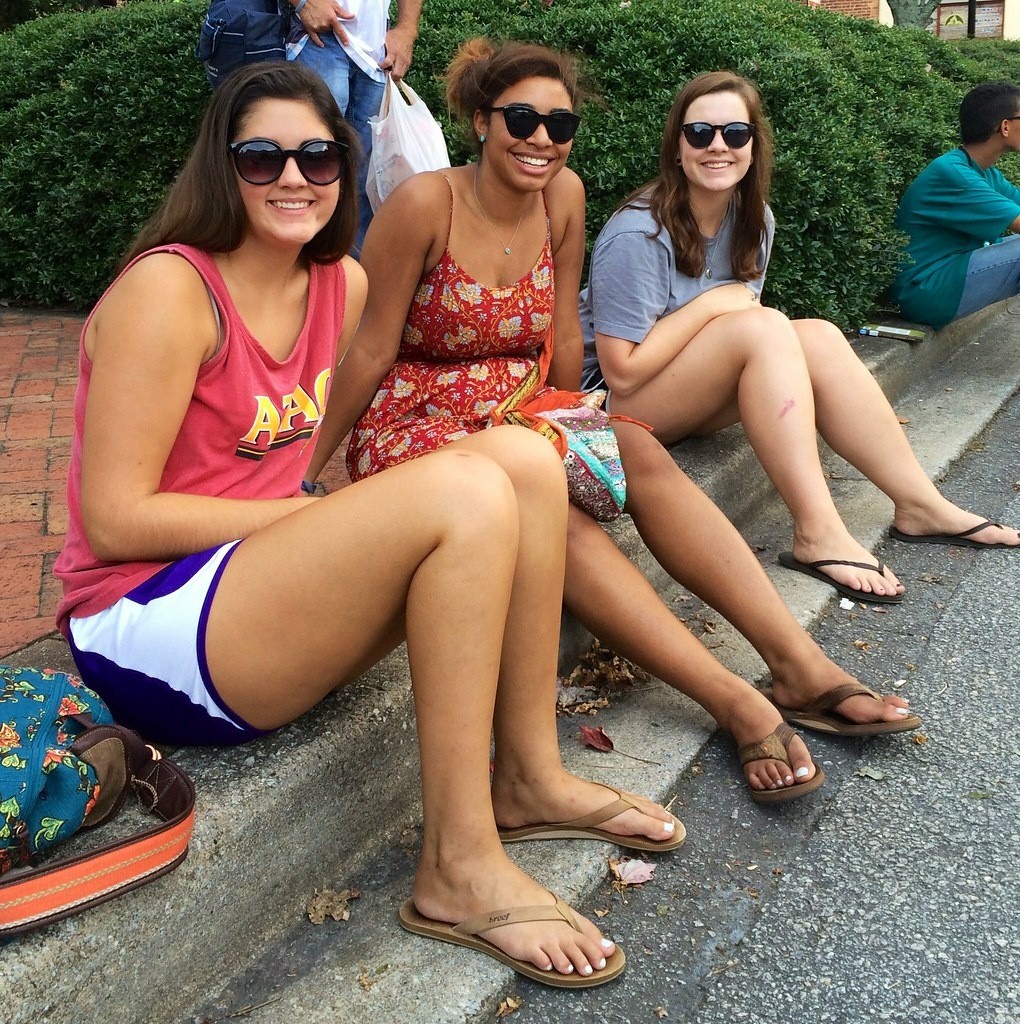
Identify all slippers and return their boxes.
[889,517,1020,549]
[777,551,906,604]
[398,888,626,988]
[759,683,922,736]
[725,688,825,804]
[499,780,687,850]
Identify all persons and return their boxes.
[577,73,1020,603]
[52,60,686,986]
[891,85,1020,331]
[278,0,423,262]
[300,37,920,803]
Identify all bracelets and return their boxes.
[295,0,306,12]
[301,480,316,494]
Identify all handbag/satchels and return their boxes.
[493,323,655,524]
[193,0,292,98]
[0,665,196,939]
[364,70,451,216]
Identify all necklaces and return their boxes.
[474,164,523,254]
[705,203,729,279]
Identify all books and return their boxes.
[858,325,926,342]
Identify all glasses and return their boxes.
[488,105,582,144]
[680,121,756,148]
[226,137,352,186]
